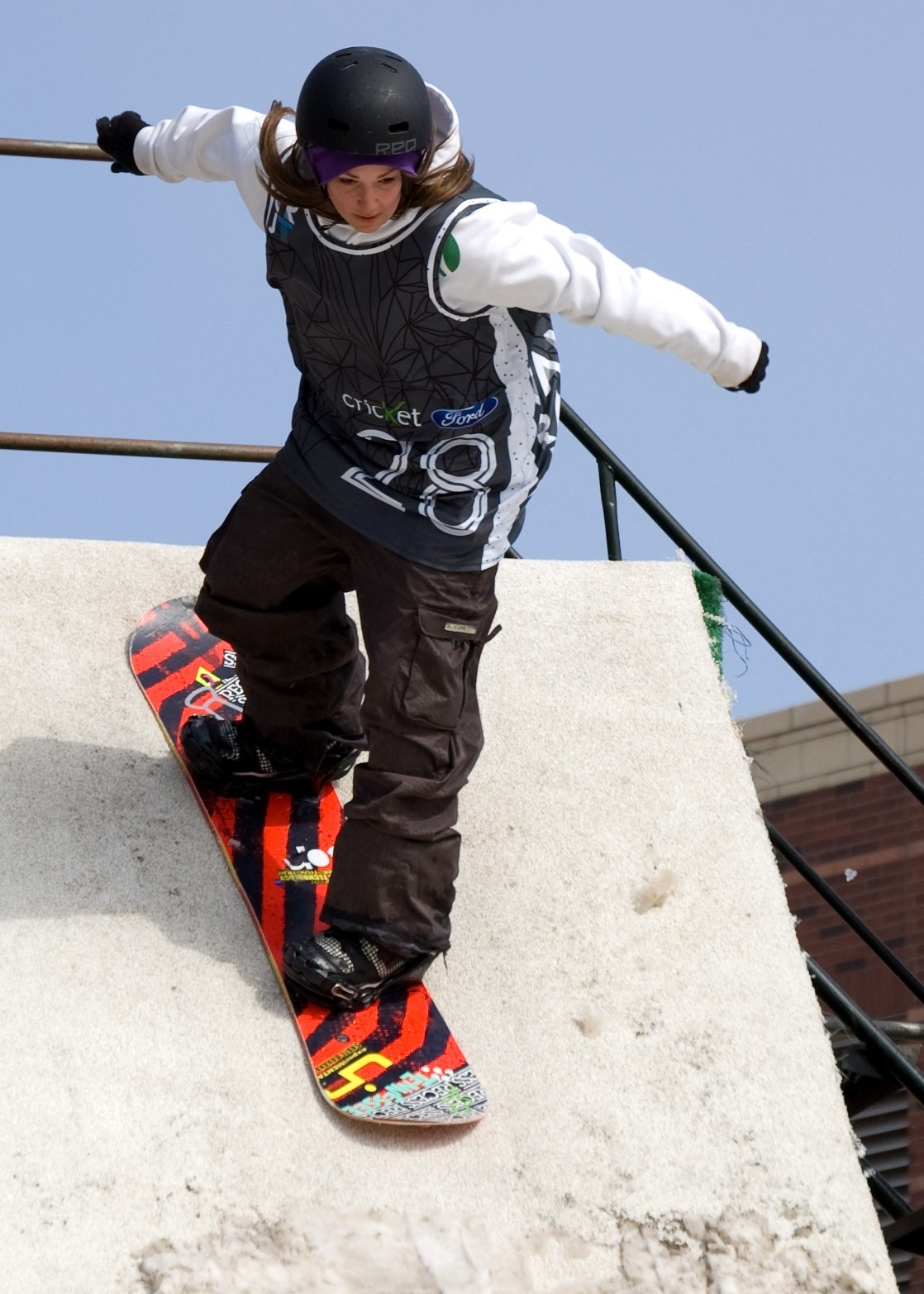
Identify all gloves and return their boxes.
[95,110,154,175]
[728,342,769,393]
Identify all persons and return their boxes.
[93,47,769,1008]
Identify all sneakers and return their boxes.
[282,922,436,1007]
[181,714,358,786]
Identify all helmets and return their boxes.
[295,49,433,159]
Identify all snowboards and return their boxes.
[126,592,490,1128]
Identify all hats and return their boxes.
[305,140,424,186]
